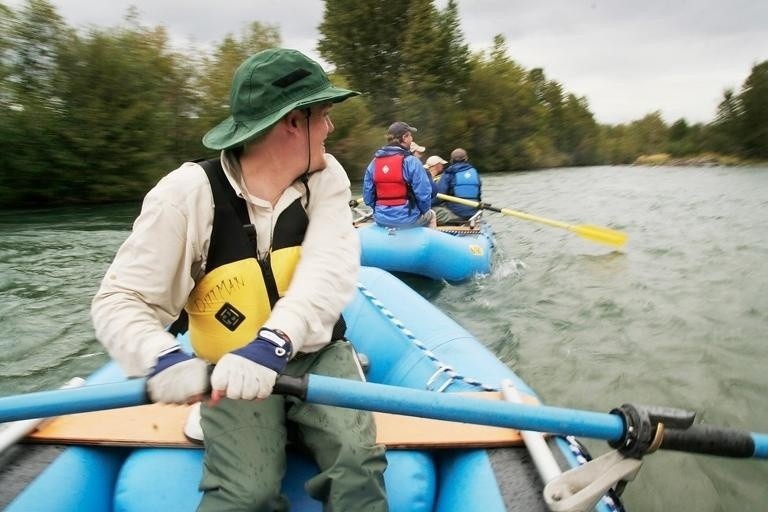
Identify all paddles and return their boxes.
[437,192,628,248]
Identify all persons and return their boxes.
[360,117,482,229]
[90,46,388,512]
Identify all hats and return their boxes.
[409,141,426,154]
[201,49,362,151]
[452,148,467,163]
[386,122,417,137]
[426,155,448,167]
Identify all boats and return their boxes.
[358,211,495,285]
[1,262,626,511]
